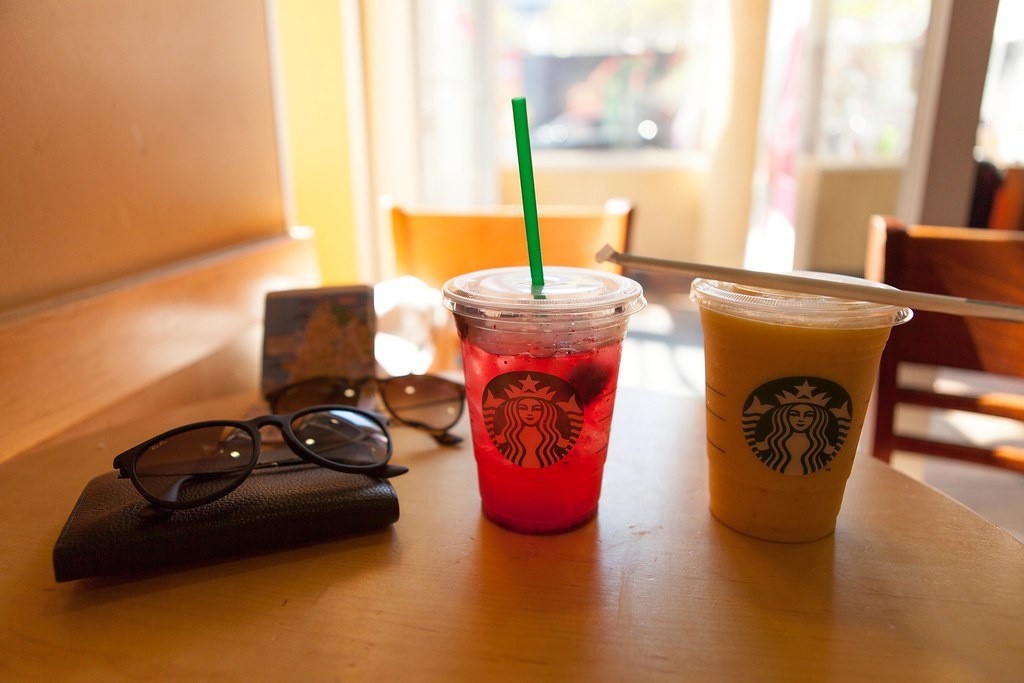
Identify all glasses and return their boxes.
[114,404,410,508]
[266,375,465,447]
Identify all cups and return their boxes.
[687,269,914,545]
[443,266,648,533]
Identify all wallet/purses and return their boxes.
[53,445,399,583]
